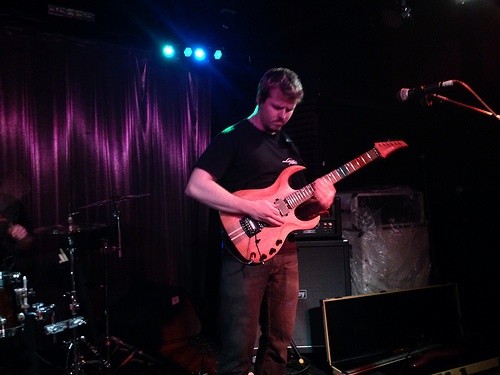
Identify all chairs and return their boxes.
[0,207,39,263]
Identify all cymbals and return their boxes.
[32,224,107,234]
[0,193,24,230]
[79,193,152,209]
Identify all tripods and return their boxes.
[64,201,160,375]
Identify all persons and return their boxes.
[186,67,337,375]
[0,217,35,256]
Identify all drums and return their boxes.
[0,270,28,339]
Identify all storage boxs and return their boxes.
[337,189,426,232]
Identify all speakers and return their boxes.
[287,240,352,349]
[343,225,430,296]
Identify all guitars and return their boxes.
[219,139,408,266]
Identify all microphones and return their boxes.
[396,80,456,104]
